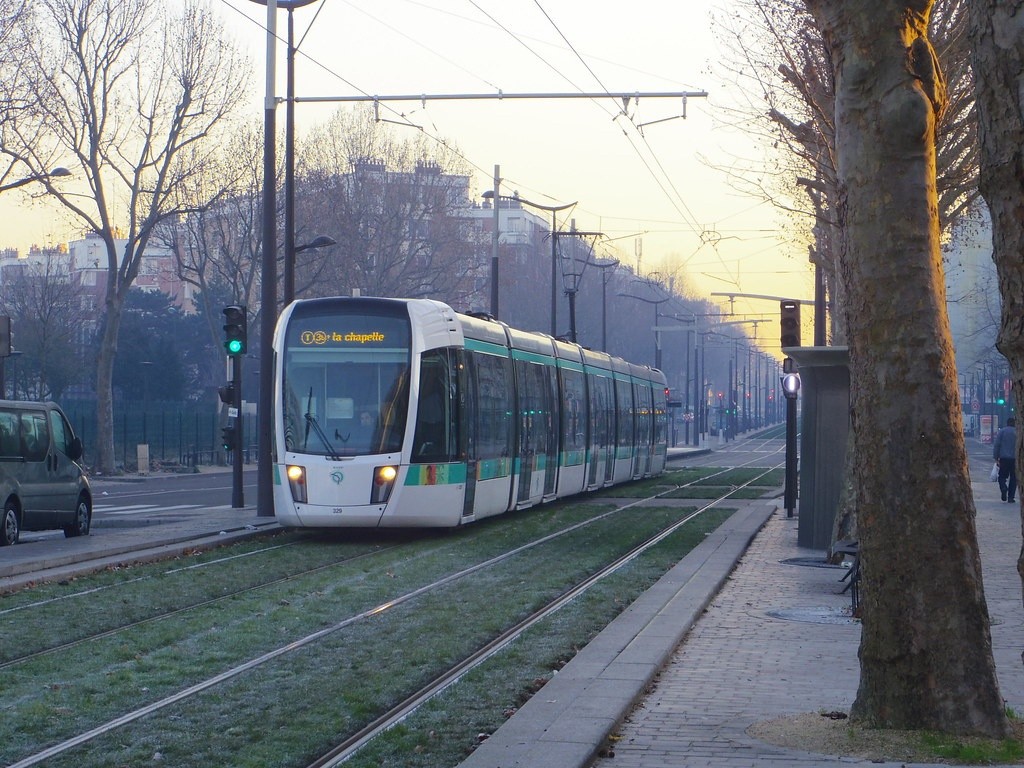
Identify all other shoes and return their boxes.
[1008,498,1015,503]
[1001,489,1007,501]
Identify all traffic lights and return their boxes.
[221,427,234,451]
[725,409,730,414]
[223,305,248,355]
[747,393,750,398]
[732,409,737,415]
[665,387,670,395]
[717,392,723,398]
[770,389,774,393]
[998,390,1005,406]
[769,395,773,400]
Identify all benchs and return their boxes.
[831,540,859,617]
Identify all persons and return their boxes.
[993,417,1018,504]
[351,410,384,443]
[21,420,36,446]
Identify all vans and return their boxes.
[0,399,93,545]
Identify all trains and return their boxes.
[269,231,667,528]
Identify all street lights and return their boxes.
[967,372,975,399]
[658,312,700,443]
[976,360,986,415]
[617,293,670,368]
[959,373,966,413]
[481,191,577,339]
[706,329,785,442]
[557,253,620,353]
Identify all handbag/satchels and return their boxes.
[989,462,1000,483]
